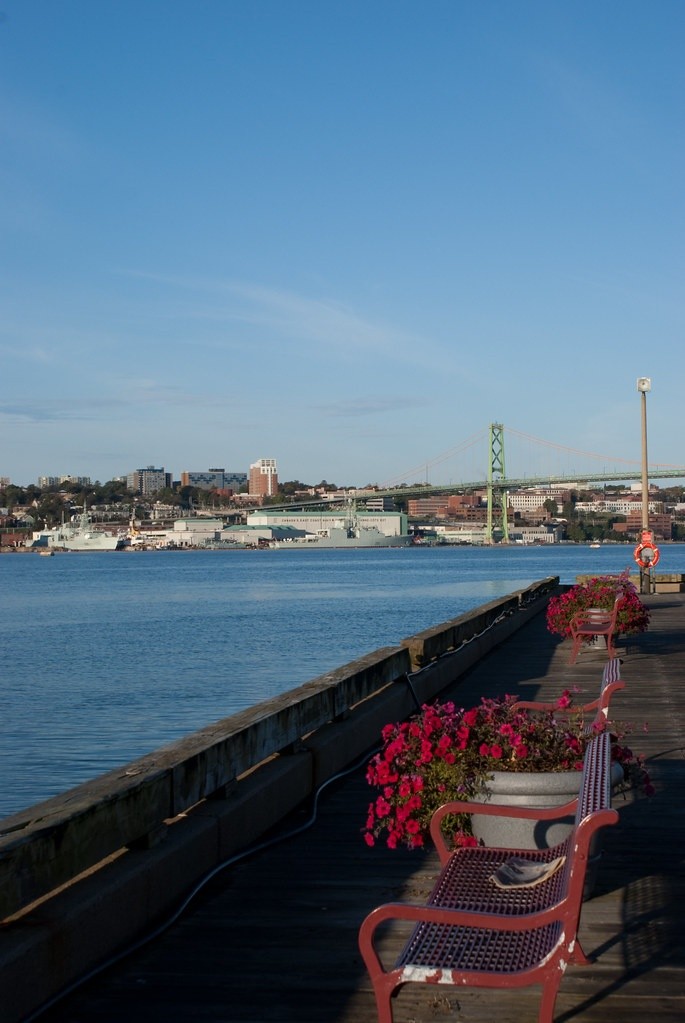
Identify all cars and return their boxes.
[129,535,151,546]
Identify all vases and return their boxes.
[465,756,624,906]
[580,606,616,650]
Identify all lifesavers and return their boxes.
[634,541,660,568]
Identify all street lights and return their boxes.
[636,377,658,594]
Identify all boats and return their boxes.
[155,544,189,550]
[198,539,250,549]
[49,500,125,551]
[590,544,601,549]
[270,498,416,549]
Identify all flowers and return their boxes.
[356,684,657,846]
[544,574,651,640]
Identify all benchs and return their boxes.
[567,592,625,665]
[358,731,623,1023]
[508,657,626,727]
